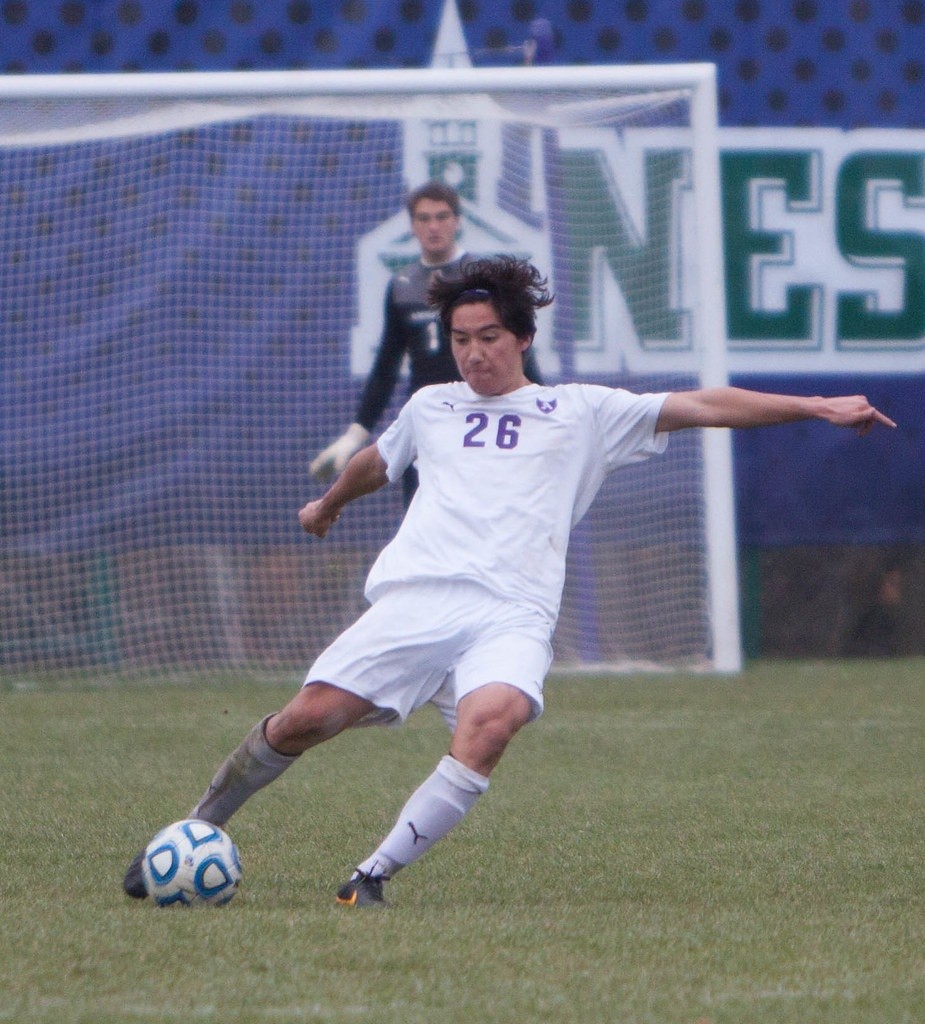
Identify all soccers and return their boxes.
[140,819,242,909]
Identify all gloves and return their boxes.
[309,422,370,481]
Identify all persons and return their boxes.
[124,253,898,909]
[309,181,545,513]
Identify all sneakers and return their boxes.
[335,861,391,908]
[123,847,149,899]
